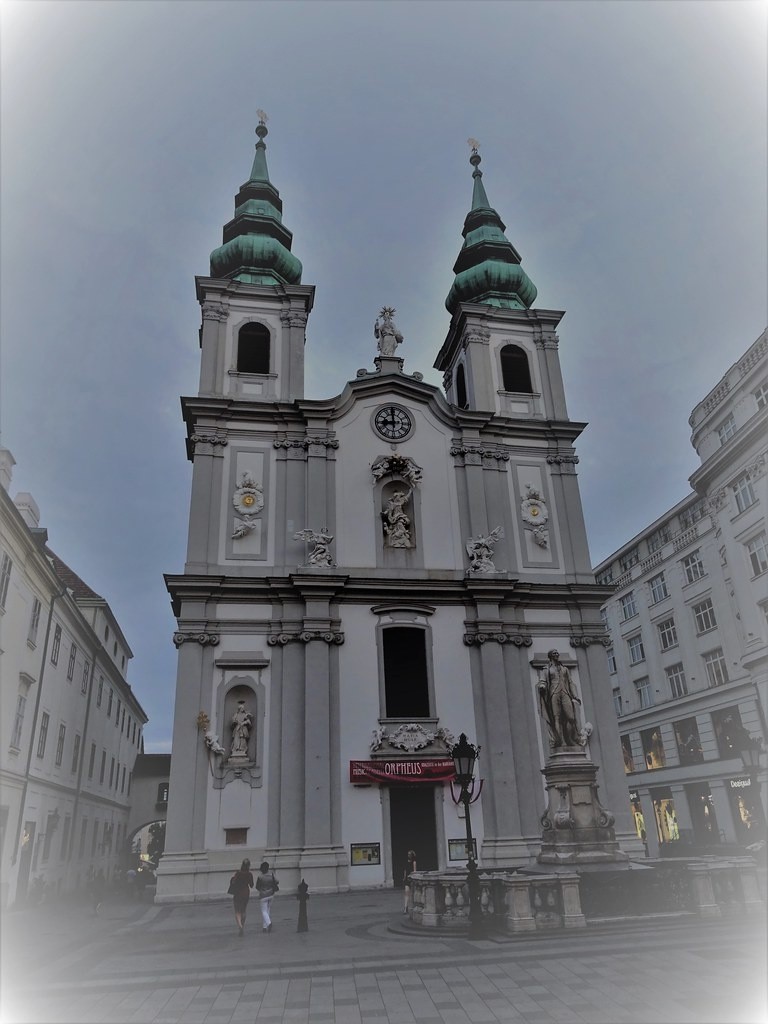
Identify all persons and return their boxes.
[231,515,256,538]
[382,484,418,547]
[533,525,549,548]
[403,850,417,913]
[434,728,455,754]
[535,648,582,746]
[292,527,335,567]
[92,864,148,913]
[229,858,254,929]
[230,705,254,758]
[256,862,279,930]
[204,731,225,756]
[369,726,388,750]
[374,306,404,357]
[466,525,504,573]
[579,722,593,746]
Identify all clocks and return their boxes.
[370,402,416,443]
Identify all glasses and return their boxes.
[407,854,411,855]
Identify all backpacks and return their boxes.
[228,871,242,894]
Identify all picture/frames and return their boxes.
[350,842,381,866]
[448,838,478,861]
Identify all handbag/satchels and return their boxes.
[272,872,279,891]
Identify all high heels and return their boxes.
[403,906,408,914]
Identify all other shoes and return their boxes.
[263,928,266,933]
[269,924,272,932]
[238,926,243,936]
